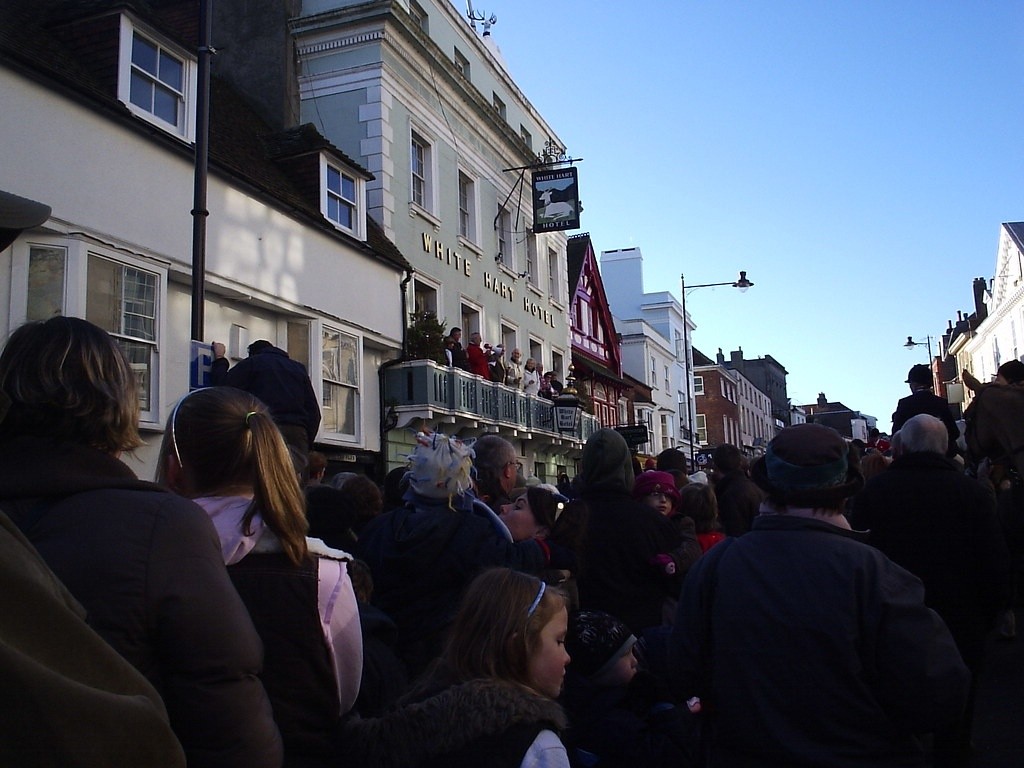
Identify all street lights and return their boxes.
[681,271,755,474]
[903,334,936,395]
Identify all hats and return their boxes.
[905,364,932,386]
[634,471,682,518]
[397,427,477,509]
[751,423,865,503]
[565,608,638,678]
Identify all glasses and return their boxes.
[501,462,522,471]
[640,491,673,501]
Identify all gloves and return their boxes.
[646,553,677,588]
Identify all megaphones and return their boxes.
[484,343,502,356]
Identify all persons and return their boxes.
[0,316,284,768]
[157,386,363,768]
[1,509,186,768]
[443,327,563,400]
[211,340,1024,768]
[344,567,571,768]
